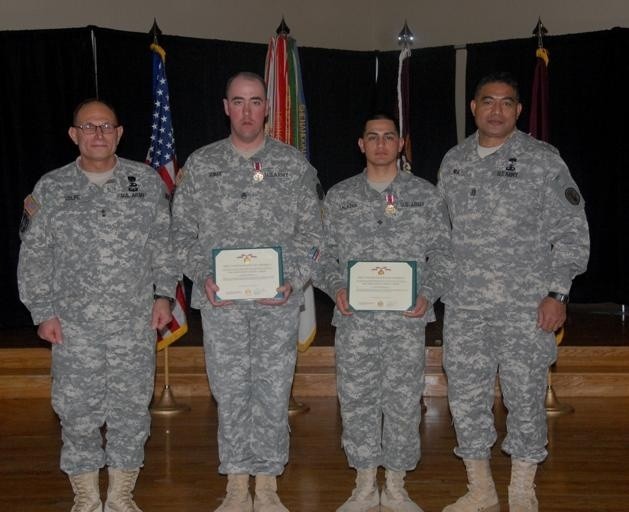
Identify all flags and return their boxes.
[263,16,319,353]
[140,16,190,353]
[525,15,565,373]
[394,21,414,180]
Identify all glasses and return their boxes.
[72,124,119,134]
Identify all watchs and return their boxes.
[546,292,568,304]
[153,294,175,303]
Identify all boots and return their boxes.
[381,469,423,512]
[508,459,539,512]
[213,474,253,511]
[336,468,380,512]
[442,459,500,512]
[69,469,102,512]
[104,467,143,512]
[253,475,290,512]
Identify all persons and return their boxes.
[436,71,591,512]
[170,69,325,512]
[16,100,177,512]
[319,109,450,511]
